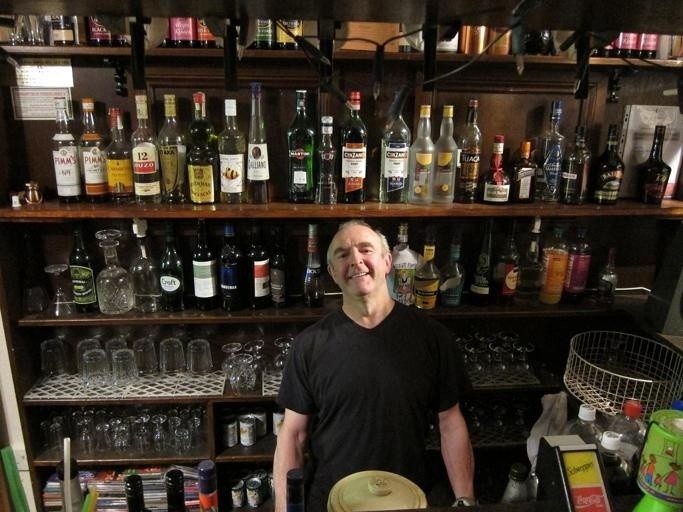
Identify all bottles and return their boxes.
[414,225,439,309]
[488,28,509,55]
[464,26,485,54]
[604,39,617,59]
[170,18,195,49]
[597,248,616,307]
[432,105,457,204]
[131,222,162,311]
[671,36,682,61]
[104,107,134,204]
[217,100,245,204]
[637,125,671,204]
[85,15,112,47]
[538,227,567,306]
[469,217,494,306]
[252,469,268,496]
[559,229,590,303]
[454,100,481,203]
[218,217,246,312]
[658,34,672,60]
[379,92,410,204]
[77,97,108,204]
[438,232,463,307]
[245,222,270,309]
[407,106,434,204]
[617,33,639,59]
[123,474,150,512]
[639,34,657,60]
[314,116,337,205]
[20,229,52,302]
[287,90,315,204]
[157,94,187,205]
[160,224,184,312]
[186,93,217,205]
[269,226,289,310]
[276,19,298,50]
[493,220,518,307]
[197,19,218,49]
[191,217,219,311]
[612,400,646,493]
[592,125,623,206]
[229,479,246,509]
[239,415,255,446]
[197,460,218,511]
[272,409,285,435]
[561,128,591,206]
[398,23,425,52]
[113,33,131,47]
[246,479,262,507]
[130,96,161,205]
[69,230,100,315]
[338,92,367,204]
[508,141,536,204]
[434,23,459,52]
[56,458,83,512]
[51,14,79,47]
[516,219,543,298]
[386,224,417,306]
[252,407,266,438]
[51,97,83,205]
[560,404,602,451]
[246,83,271,204]
[302,224,324,307]
[254,20,274,50]
[478,135,510,205]
[97,228,134,315]
[535,100,564,202]
[165,469,186,512]
[597,431,629,512]
[497,463,528,504]
[221,416,238,448]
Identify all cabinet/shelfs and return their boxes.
[0,0,683,512]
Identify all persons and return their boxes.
[272,222,476,510]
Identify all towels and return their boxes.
[526,392,567,475]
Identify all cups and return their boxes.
[243,341,266,372]
[485,343,508,382]
[40,339,70,377]
[110,347,140,388]
[39,406,208,455]
[105,337,129,363]
[159,339,185,374]
[474,328,497,350]
[219,342,243,375]
[425,389,532,439]
[131,338,159,375]
[229,354,257,395]
[44,262,75,319]
[186,339,212,377]
[22,286,51,315]
[508,340,535,382]
[54,325,77,352]
[453,333,473,352]
[463,343,484,382]
[79,325,267,341]
[81,348,111,391]
[497,328,524,347]
[76,337,103,378]
[272,335,295,378]
